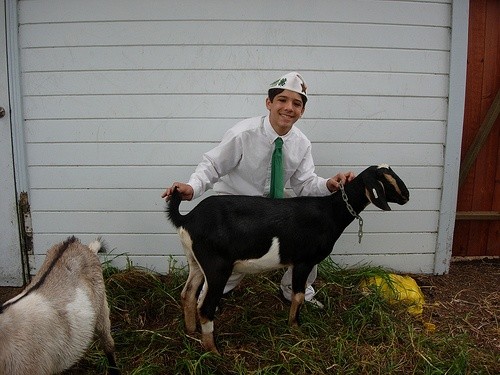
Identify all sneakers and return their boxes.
[302,297,323,310]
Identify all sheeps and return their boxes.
[163,163,410,357]
[0,235,122,375]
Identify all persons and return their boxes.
[161,71,355,307]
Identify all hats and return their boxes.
[267,72,308,100]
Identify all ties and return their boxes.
[270,137,285,199]
